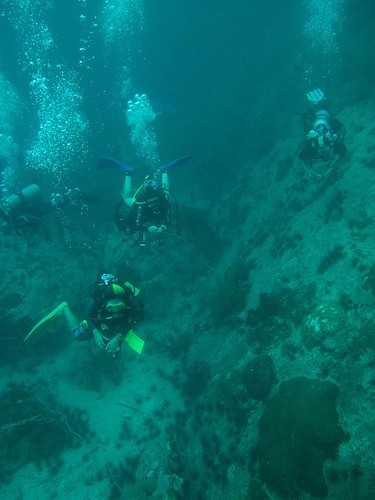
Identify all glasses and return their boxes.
[106,303,126,313]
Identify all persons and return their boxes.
[0,179,52,248]
[97,156,191,245]
[299,88,347,168]
[24,272,145,359]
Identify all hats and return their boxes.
[143,179,160,197]
[314,135,331,152]
[104,283,125,299]
